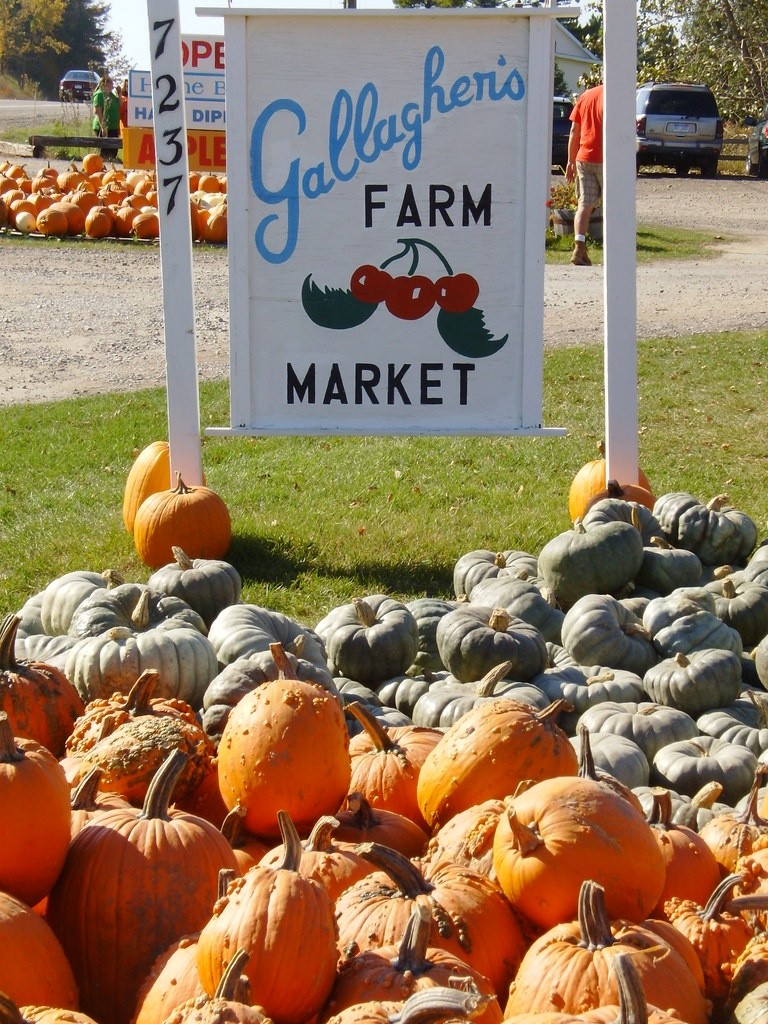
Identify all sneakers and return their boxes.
[571,240,591,265]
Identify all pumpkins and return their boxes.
[0,471,768,1023]
[0,153,227,245]
[123,441,208,535]
[569,440,650,521]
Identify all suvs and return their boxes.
[637,82,724,178]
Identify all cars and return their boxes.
[57,71,102,102]
[552,97,574,172]
[745,104,768,175]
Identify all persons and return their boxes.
[565,84,604,265]
[93,75,122,162]
[121,78,128,127]
